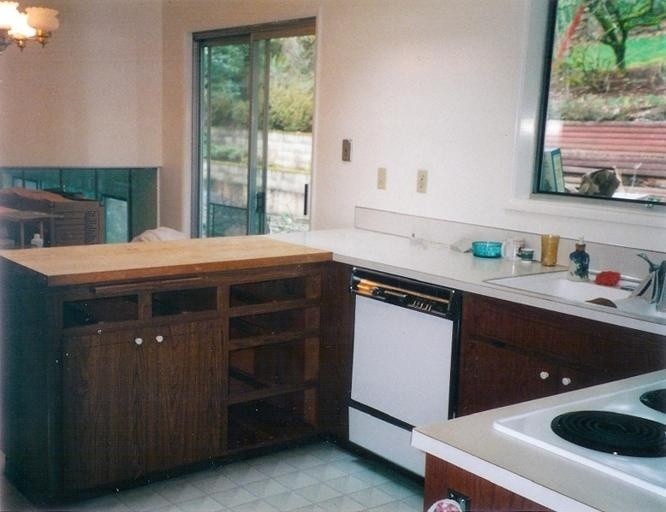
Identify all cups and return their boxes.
[540,232,560,267]
[501,237,526,262]
[516,247,534,265]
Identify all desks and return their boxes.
[0,206,64,249]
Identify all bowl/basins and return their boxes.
[471,239,503,258]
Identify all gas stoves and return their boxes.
[491,376,666,498]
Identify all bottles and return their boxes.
[567,237,591,283]
[30,233,43,249]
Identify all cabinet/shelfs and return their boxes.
[0,234,333,508]
[459,291,666,420]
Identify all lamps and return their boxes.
[0,1,59,54]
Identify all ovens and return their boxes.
[333,264,464,483]
